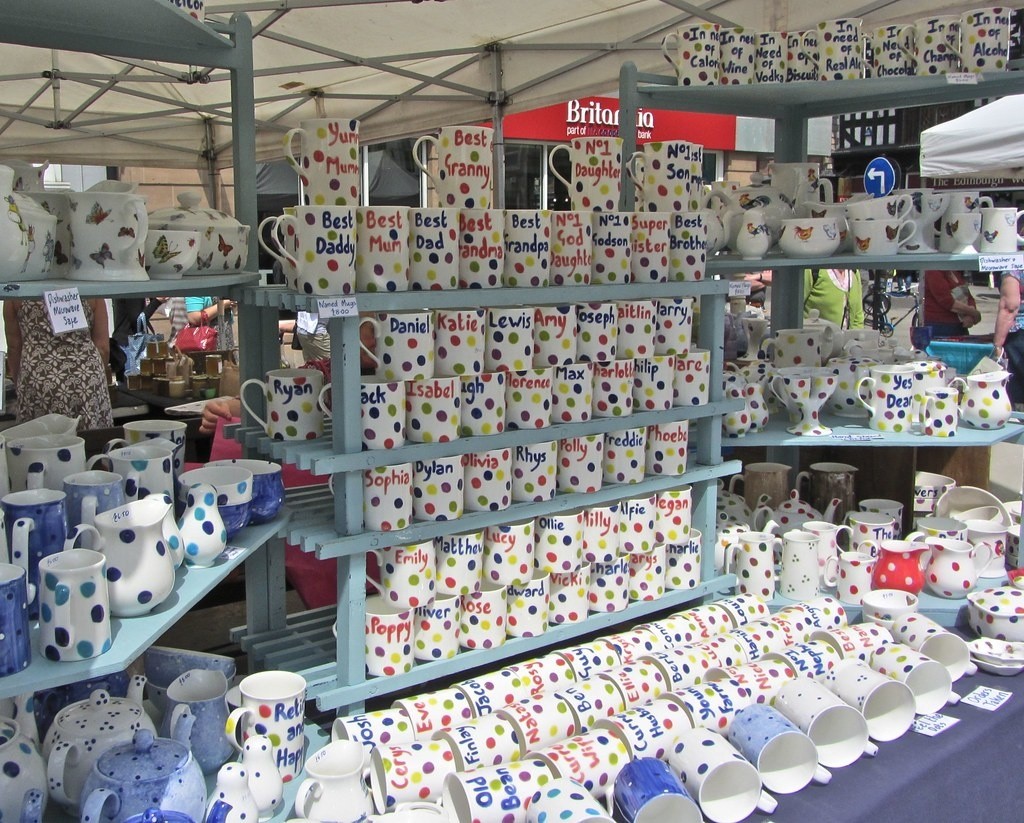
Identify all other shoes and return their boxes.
[896,288,904,293]
[906,287,911,290]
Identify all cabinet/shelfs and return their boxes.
[0,2,294,699]
[616,59,1024,627]
[222,279,746,718]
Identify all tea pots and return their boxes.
[0,667,207,822]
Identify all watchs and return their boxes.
[758,273,763,284]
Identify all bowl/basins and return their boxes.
[140,646,234,685]
[0,158,251,283]
[143,669,235,717]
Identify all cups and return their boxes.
[262,118,707,296]
[327,417,687,533]
[169,381,186,398]
[661,6,1024,86]
[708,163,1024,254]
[238,297,712,450]
[332,487,700,681]
[719,326,1013,440]
[0,412,285,678]
[225,462,1023,822]
[169,0,206,25]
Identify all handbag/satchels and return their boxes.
[218,345,240,397]
[166,344,194,389]
[174,310,217,351]
[128,311,165,377]
[968,343,1009,377]
[291,324,302,349]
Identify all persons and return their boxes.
[924,269,981,336]
[3,297,112,433]
[295,310,330,362]
[112,296,238,385]
[201,311,378,436]
[743,270,773,311]
[804,269,864,330]
[993,235,1024,404]
